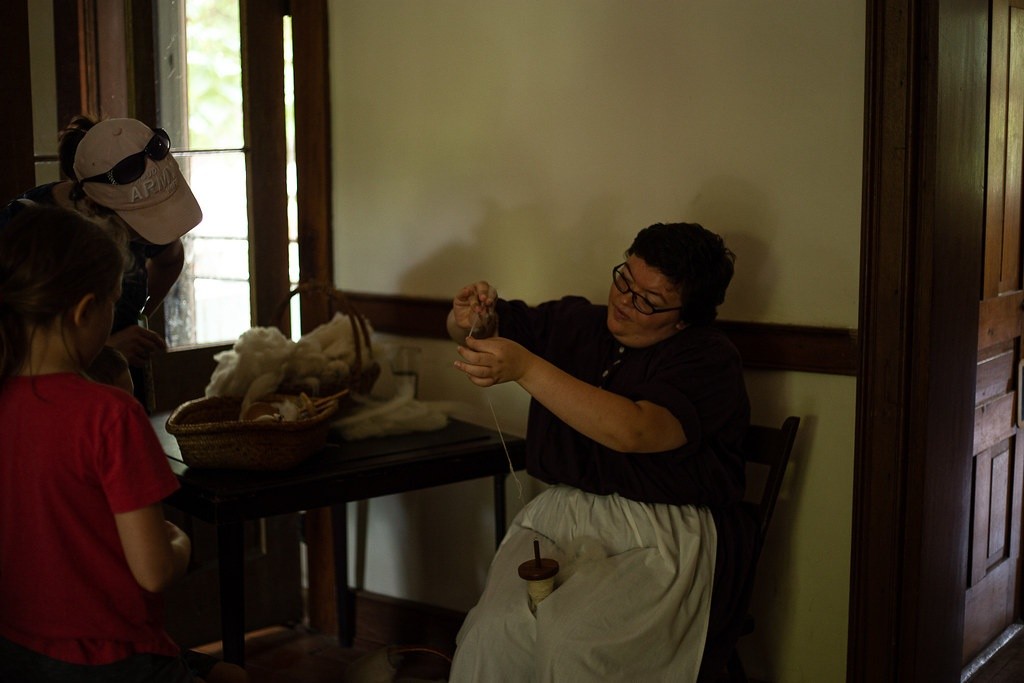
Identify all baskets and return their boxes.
[263,283,380,395]
[166,394,339,470]
[343,642,453,683]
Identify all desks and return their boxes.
[148,395,526,670]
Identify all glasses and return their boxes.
[81,128,171,186]
[612,262,683,315]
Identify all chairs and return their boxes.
[727,417,800,683]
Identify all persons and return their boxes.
[0,199,249,683]
[446,222,754,683]
[13,114,204,414]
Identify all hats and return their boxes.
[73,117,202,245]
